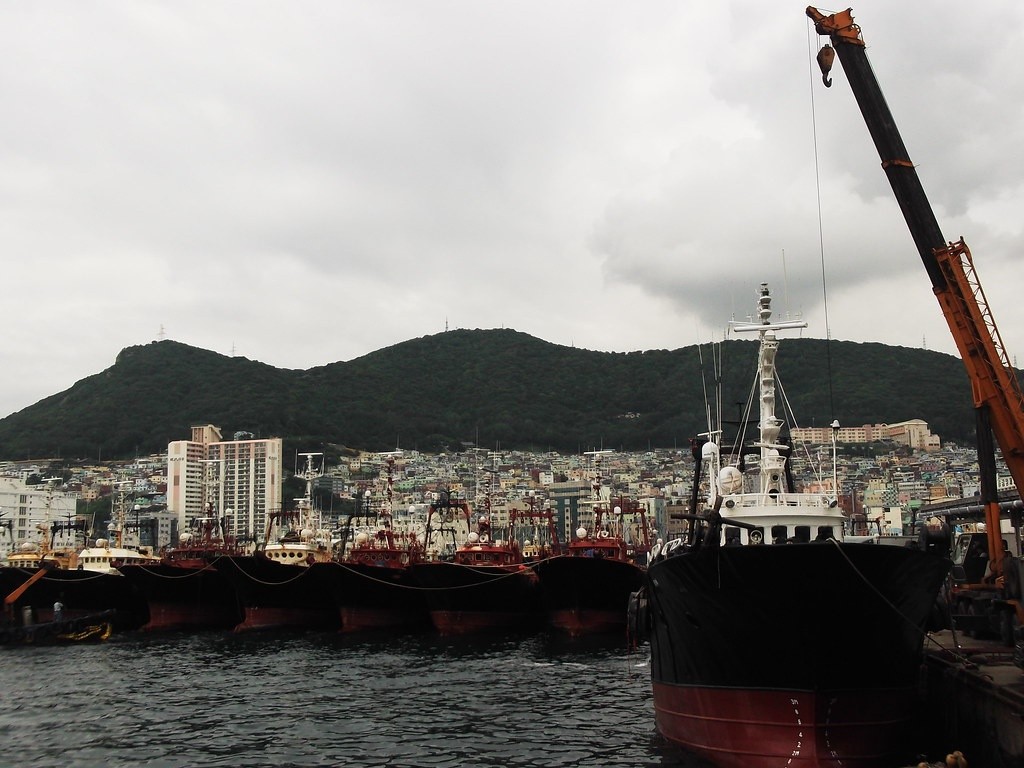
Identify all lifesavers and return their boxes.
[480,535,487,542]
[370,554,377,561]
[601,531,609,537]
[378,554,383,562]
[383,554,390,561]
[364,554,370,561]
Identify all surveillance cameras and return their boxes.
[832,423,840,431]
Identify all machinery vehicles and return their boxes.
[805,5,1024,654]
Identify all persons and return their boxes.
[776,437,794,493]
[290,520,296,532]
[949,533,1024,640]
[593,549,603,558]
[657,537,663,548]
[723,536,744,546]
[876,516,887,535]
[774,536,806,544]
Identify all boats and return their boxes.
[642,283,955,768]
[0,497,652,646]
